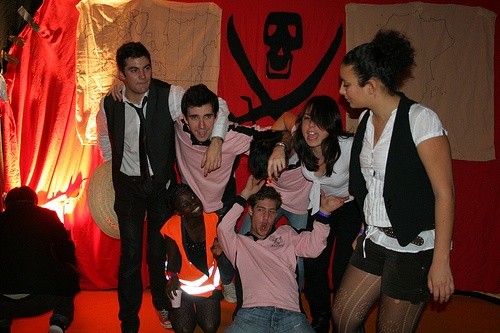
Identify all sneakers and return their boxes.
[158,310,172,329]
[121,314,139,333]
[223,282,237,303]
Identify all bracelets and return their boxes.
[276,142,285,147]
[360,229,364,234]
[319,207,331,218]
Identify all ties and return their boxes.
[126,96,154,193]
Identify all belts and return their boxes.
[379,226,423,247]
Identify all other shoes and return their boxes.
[49,318,66,333]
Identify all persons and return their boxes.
[158,183,234,333]
[105,80,273,304]
[236,93,364,333]
[216,175,350,333]
[95,40,232,332]
[329,28,456,333]
[0,186,82,333]
